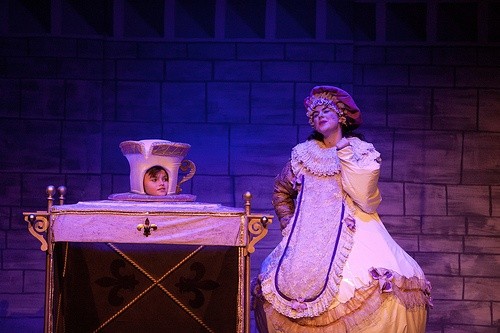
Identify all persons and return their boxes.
[249,86,433,332]
[142,165,170,197]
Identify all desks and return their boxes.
[19,203,275,333]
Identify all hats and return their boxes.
[304,85,362,130]
